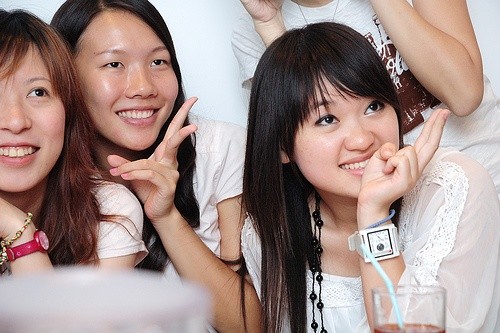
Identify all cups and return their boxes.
[371,285,447,333]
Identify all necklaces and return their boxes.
[309,192,328,333]
[297,0,339,27]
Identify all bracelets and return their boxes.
[366,208,395,229]
[0,212,33,266]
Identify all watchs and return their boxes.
[347,224,399,263]
[7,228,49,262]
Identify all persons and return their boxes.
[0,8,149,276]
[228,0,499,333]
[50,0,263,332]
